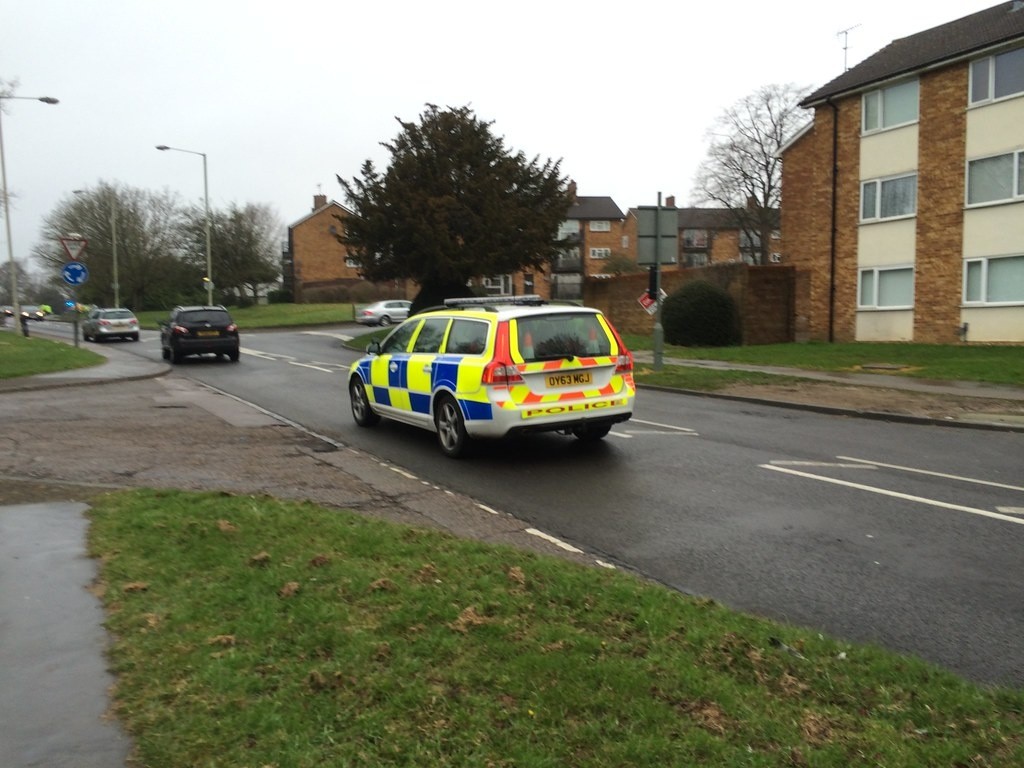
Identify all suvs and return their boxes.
[155,303,239,363]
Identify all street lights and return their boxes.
[72,187,119,309]
[0,96,59,336]
[154,142,213,307]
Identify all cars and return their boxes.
[354,299,412,329]
[18,304,45,323]
[0,305,15,318]
[79,307,139,342]
[346,293,639,457]
